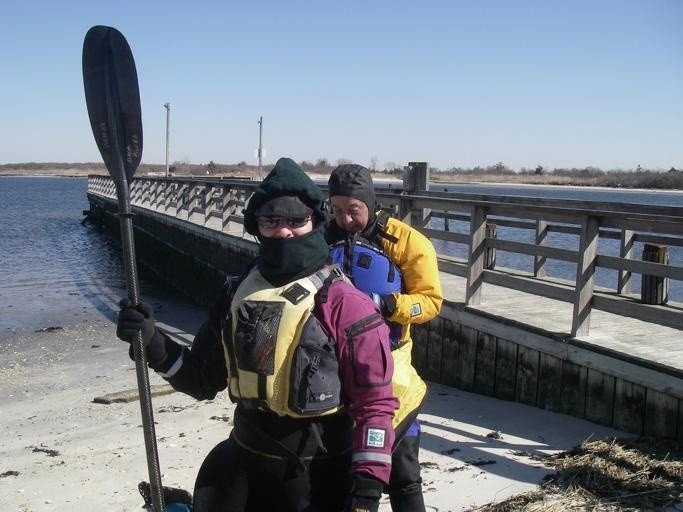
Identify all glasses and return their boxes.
[258,216,315,229]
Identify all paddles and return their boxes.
[81,24,166,511]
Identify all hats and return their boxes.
[327,164,376,228]
[253,196,313,218]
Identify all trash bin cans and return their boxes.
[485,224,496,269]
[641,244,668,304]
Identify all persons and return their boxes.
[114,157,400,511]
[319,163,446,511]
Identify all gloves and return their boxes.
[115,297,154,349]
[350,474,384,512]
[370,292,397,318]
